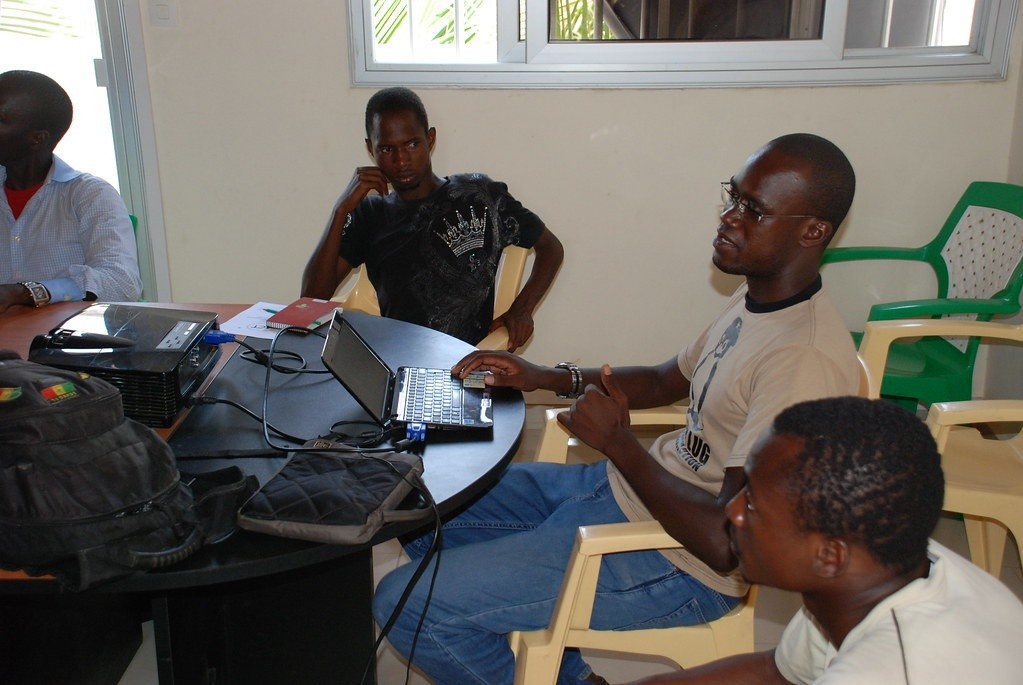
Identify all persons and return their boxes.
[300,87,565,353]
[0,70,145,314]
[371,126,869,684]
[626,396,1022,684]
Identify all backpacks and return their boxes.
[0,349,205,594]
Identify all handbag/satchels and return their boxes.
[235,438,434,545]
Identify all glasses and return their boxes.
[720,182,815,224]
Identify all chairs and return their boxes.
[817,181,1023,520]
[856,318,1023,580]
[330,243,528,350]
[504,352,874,685]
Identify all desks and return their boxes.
[0,301,525,685]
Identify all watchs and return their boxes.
[555,361,584,399]
[17,281,49,308]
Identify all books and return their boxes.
[266,297,344,334]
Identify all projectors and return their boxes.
[26,302,224,428]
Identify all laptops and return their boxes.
[321,310,494,433]
